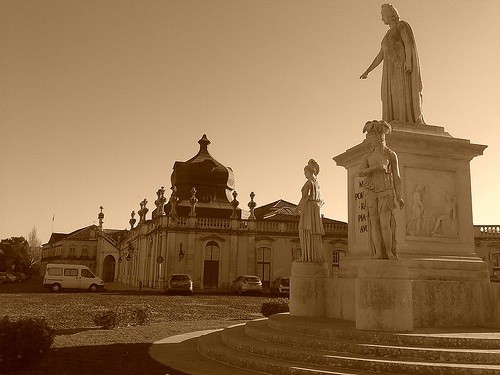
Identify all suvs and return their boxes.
[230,275,263,296]
[166,274,193,293]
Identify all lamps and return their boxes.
[179,250,185,259]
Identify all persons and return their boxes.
[430,192,457,233]
[294,158,326,261]
[359,3,426,124]
[405,182,425,236]
[358,119,405,260]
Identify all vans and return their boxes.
[43,264,105,293]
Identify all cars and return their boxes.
[271,276,291,297]
